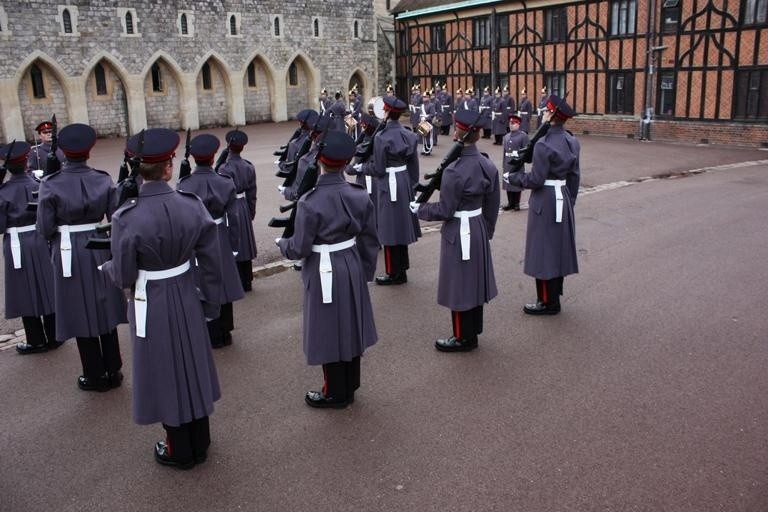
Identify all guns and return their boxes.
[213,126,239,172]
[84,129,146,262]
[179,127,193,177]
[25,114,59,256]
[347,98,398,165]
[413,109,488,203]
[269,110,334,238]
[0,139,16,189]
[507,91,570,173]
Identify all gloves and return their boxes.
[278,184,286,195]
[409,196,421,214]
[353,163,363,173]
[96,264,104,272]
[510,150,520,158]
[502,171,511,183]
[32,170,44,179]
[274,237,282,245]
[274,160,281,165]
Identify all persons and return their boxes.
[417,92,435,156]
[502,94,579,315]
[1,140,65,355]
[216,129,257,291]
[409,85,550,145]
[408,109,500,353]
[114,149,144,203]
[98,127,222,470]
[275,129,381,408]
[277,88,421,285]
[34,123,124,392]
[176,134,245,348]
[28,120,66,171]
[501,116,530,210]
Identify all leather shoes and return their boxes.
[502,204,514,211]
[210,331,234,350]
[305,388,354,410]
[433,334,479,353]
[241,281,253,293]
[421,150,432,156]
[154,439,209,471]
[106,369,124,389]
[77,374,110,392]
[523,301,561,315]
[15,339,50,356]
[293,261,304,271]
[47,336,66,351]
[375,270,407,286]
[514,205,520,211]
[438,127,504,146]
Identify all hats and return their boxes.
[506,114,523,124]
[225,130,249,147]
[320,81,549,98]
[0,140,31,165]
[453,110,489,134]
[539,95,574,123]
[58,123,99,159]
[188,133,221,161]
[382,94,408,114]
[315,131,356,167]
[34,121,54,134]
[295,108,337,136]
[126,128,181,163]
